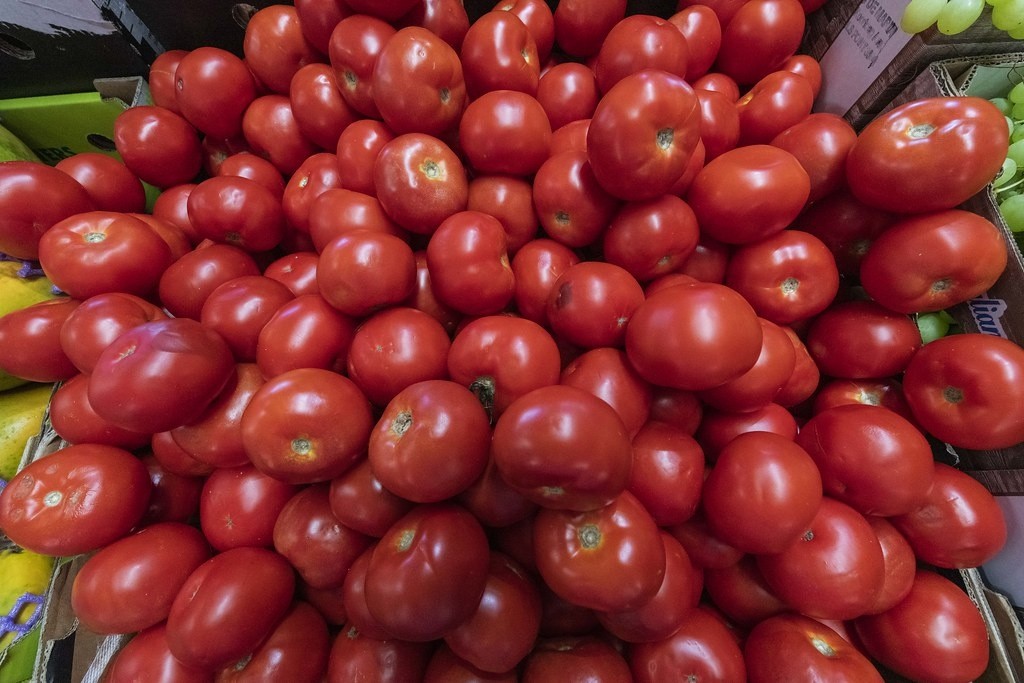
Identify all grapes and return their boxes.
[984,81,1024,233]
[900,0,1024,40]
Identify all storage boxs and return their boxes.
[0,75,151,683]
[803,1,1024,497]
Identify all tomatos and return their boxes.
[0,0,1024,683]
